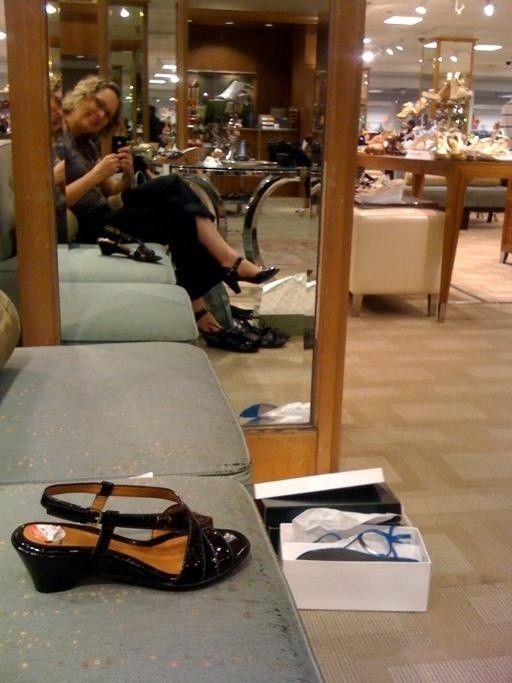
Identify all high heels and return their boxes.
[220,255,280,293]
[354,169,406,208]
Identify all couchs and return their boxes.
[1,137,201,345]
[1,291,324,682]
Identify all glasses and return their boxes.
[92,96,113,124]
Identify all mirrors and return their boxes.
[1,2,20,314]
[107,0,144,160]
[439,41,472,131]
[47,1,332,431]
[420,40,438,130]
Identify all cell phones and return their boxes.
[111,135,127,160]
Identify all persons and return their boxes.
[50,74,67,245]
[149,103,165,147]
[60,73,279,339]
[402,118,415,141]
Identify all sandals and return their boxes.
[294,523,420,561]
[195,306,290,351]
[97,225,162,262]
[362,103,511,166]
[12,480,250,592]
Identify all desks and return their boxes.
[358,150,512,322]
[152,159,324,270]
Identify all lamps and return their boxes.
[415,0,428,15]
[481,1,495,17]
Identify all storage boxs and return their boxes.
[258,483,401,556]
[280,522,430,613]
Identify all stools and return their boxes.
[349,205,446,319]
